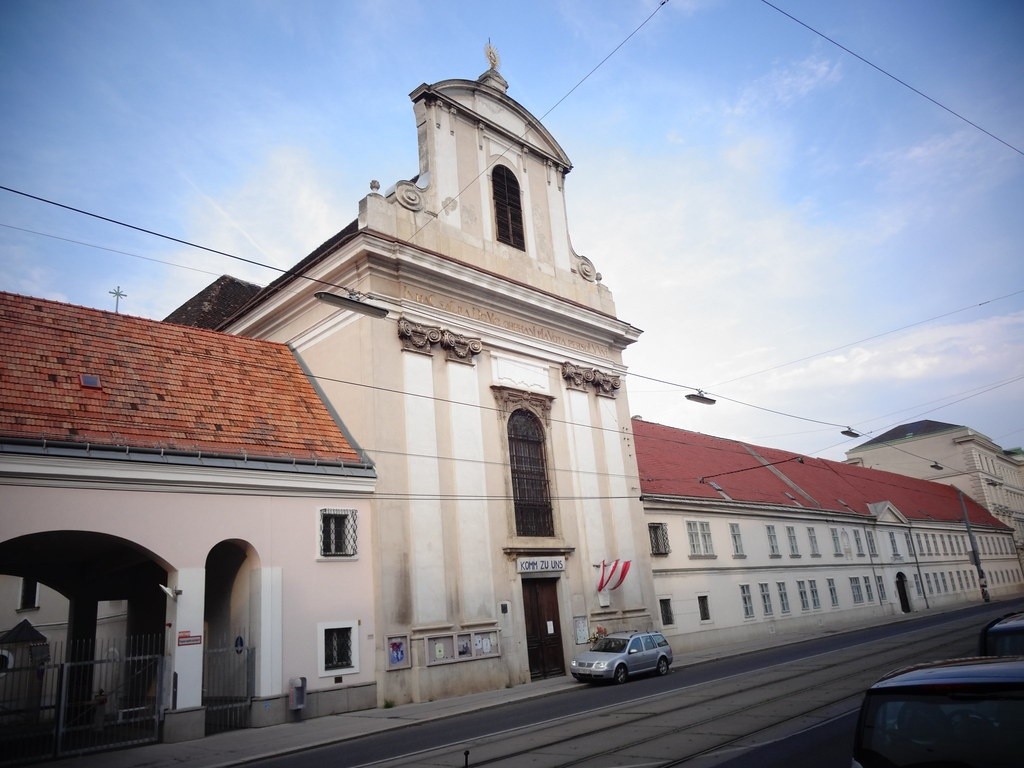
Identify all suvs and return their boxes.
[569,630,674,685]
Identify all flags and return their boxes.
[597,559,632,593]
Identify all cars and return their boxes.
[978,610,1024,656]
[849,653,1023,768]
